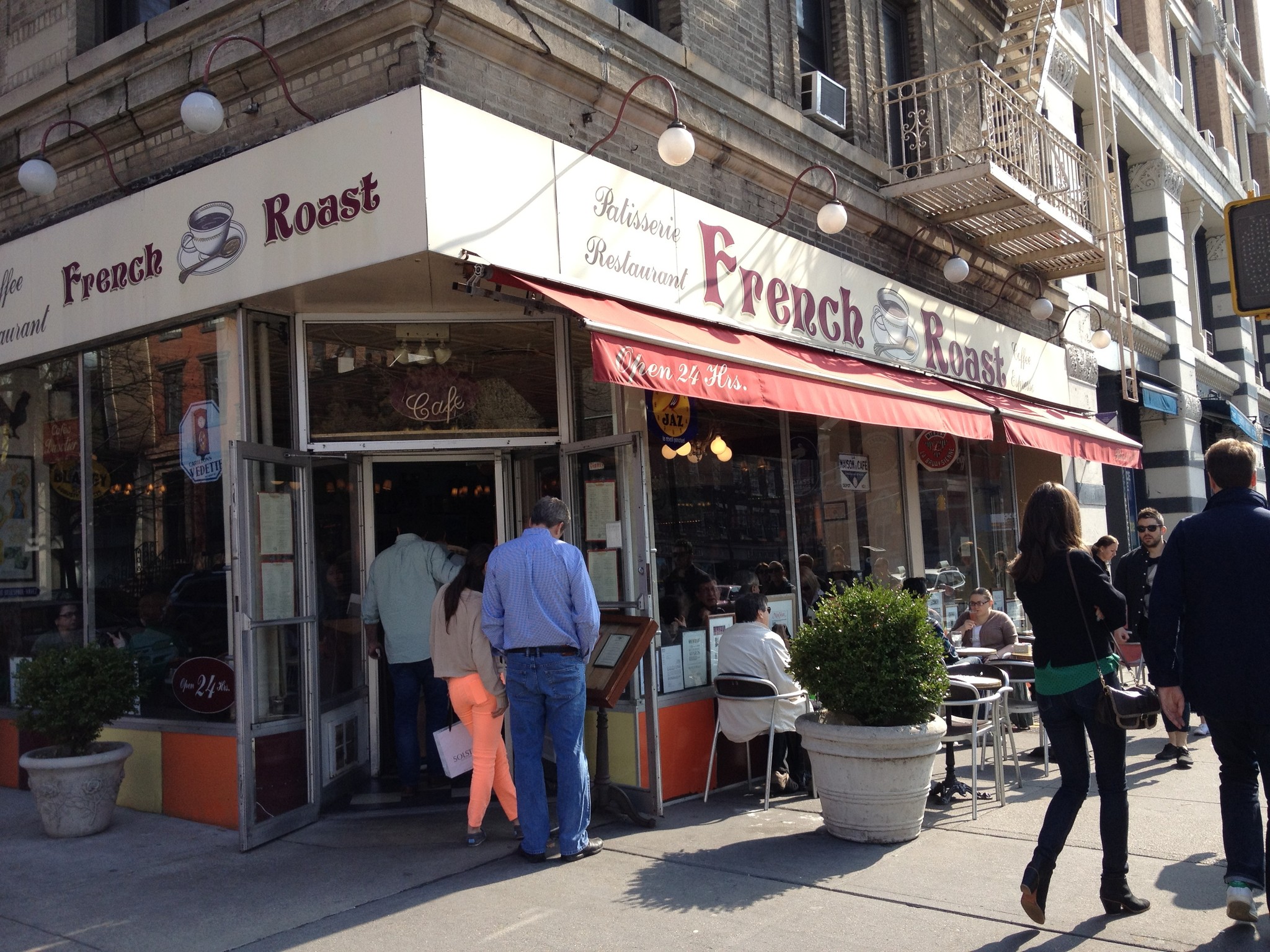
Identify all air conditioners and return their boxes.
[1198,128,1216,155]
[1174,75,1184,110]
[1243,179,1260,197]
[800,70,847,134]
[1102,0,1118,26]
[1226,24,1241,50]
[1202,328,1214,356]
[1118,269,1140,307]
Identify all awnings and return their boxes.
[1201,397,1264,444]
[937,377,1145,473]
[478,266,996,443]
[1096,368,1182,415]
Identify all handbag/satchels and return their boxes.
[1094,684,1162,730]
[433,693,474,778]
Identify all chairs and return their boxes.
[696,624,1151,820]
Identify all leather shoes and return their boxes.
[561,837,604,862]
[517,841,547,863]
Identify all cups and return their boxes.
[950,631,963,651]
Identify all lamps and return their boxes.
[391,321,455,365]
[888,223,969,283]
[767,166,848,235]
[587,73,696,167]
[1046,304,1112,349]
[655,396,733,463]
[979,270,1053,320]
[17,119,132,197]
[180,34,320,135]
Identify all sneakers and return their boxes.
[1194,723,1210,735]
[1226,880,1259,922]
[514,816,560,839]
[465,827,490,847]
[1155,743,1177,760]
[1176,746,1193,764]
[770,771,800,793]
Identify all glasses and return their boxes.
[55,611,80,620]
[756,568,783,574]
[765,607,771,614]
[672,551,691,558]
[968,600,990,606]
[1136,524,1162,533]
[801,585,810,591]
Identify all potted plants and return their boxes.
[8,639,149,835]
[783,571,959,845]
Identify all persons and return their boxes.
[1009,480,1153,926]
[314,559,355,701]
[1140,438,1270,925]
[481,494,607,863]
[166,533,229,660]
[658,536,1016,645]
[1112,507,1193,769]
[105,588,197,692]
[946,586,1019,681]
[429,540,563,849]
[1087,534,1118,581]
[1188,702,1212,738]
[716,593,818,798]
[361,509,471,801]
[32,599,83,660]
[897,578,1004,746]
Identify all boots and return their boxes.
[1099,873,1151,914]
[1020,854,1056,924]
